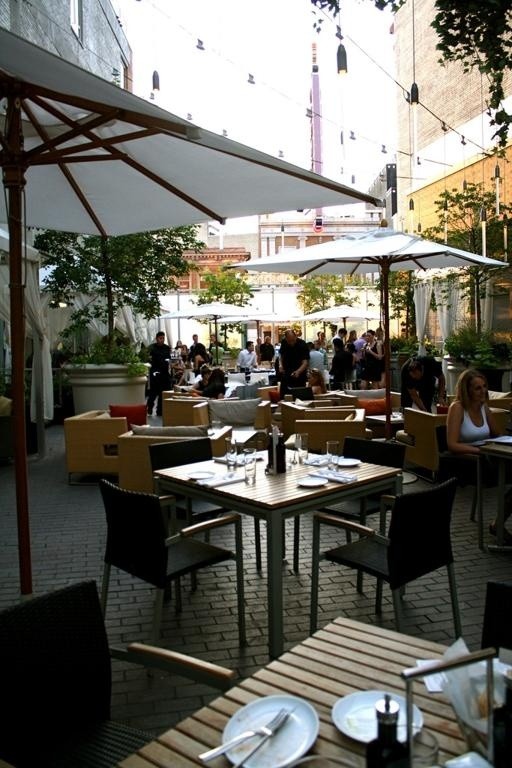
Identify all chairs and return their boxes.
[63,367,512,491]
[149,436,261,589]
[293,437,407,572]
[310,471,462,638]
[435,425,484,520]
[98,479,248,649]
[0,580,238,768]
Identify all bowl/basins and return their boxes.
[228,369,235,372]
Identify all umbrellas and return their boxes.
[209,308,303,346]
[156,299,261,364]
[219,219,509,485]
[1,25,386,599]
[289,303,390,345]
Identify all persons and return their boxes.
[446,367,511,543]
[399,356,446,415]
[146,325,409,418]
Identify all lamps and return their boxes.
[337,0,347,73]
[410,0,418,104]
[153,71,159,91]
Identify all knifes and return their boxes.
[231,709,292,768]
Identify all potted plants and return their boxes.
[387,326,512,404]
[33,220,205,416]
[395,180,511,391]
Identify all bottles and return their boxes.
[366,693,412,768]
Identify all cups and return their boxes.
[170,347,181,358]
[224,437,238,468]
[242,447,256,484]
[285,444,293,471]
[391,411,403,418]
[326,440,340,471]
[296,432,309,464]
[393,722,439,768]
[329,350,333,356]
[282,753,359,768]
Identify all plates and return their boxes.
[329,690,427,745]
[222,694,320,767]
[296,477,329,488]
[188,471,214,481]
[337,458,362,466]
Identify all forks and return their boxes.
[198,703,292,763]
